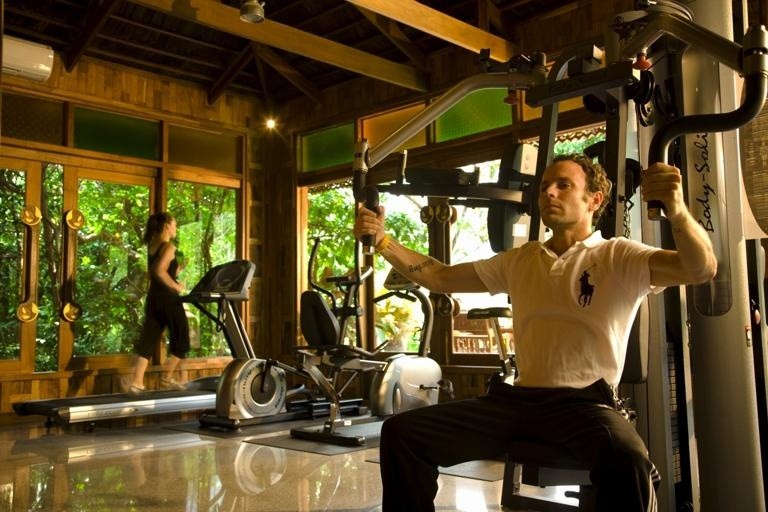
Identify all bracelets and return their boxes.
[374,234,391,253]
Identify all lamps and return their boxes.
[239,0,265,24]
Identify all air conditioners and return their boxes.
[3,33,55,83]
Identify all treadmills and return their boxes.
[13,259,257,433]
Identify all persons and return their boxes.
[129,211,189,395]
[353,154,718,512]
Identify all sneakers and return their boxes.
[127,384,151,400]
[159,378,187,392]
